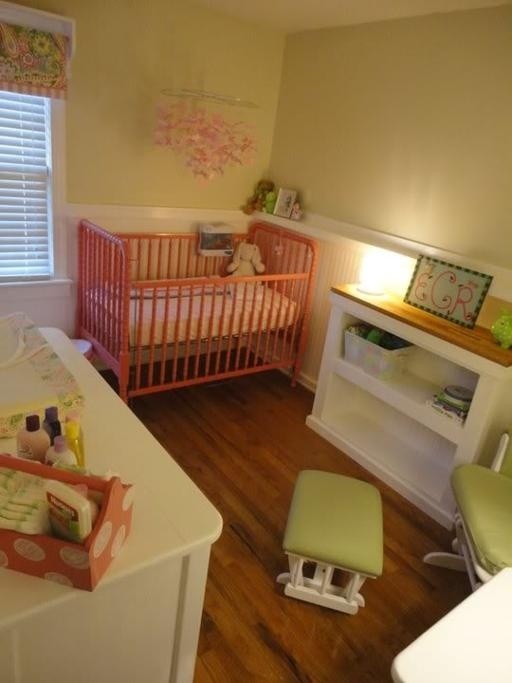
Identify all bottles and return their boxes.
[16,405,85,471]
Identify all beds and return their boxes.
[73,219,320,406]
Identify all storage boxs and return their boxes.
[0,452,131,592]
[344,324,415,380]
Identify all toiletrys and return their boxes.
[17,407,84,471]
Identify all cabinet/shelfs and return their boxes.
[304,281,512,531]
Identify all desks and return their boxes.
[0,324,224,683]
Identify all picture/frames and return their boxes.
[403,254,494,330]
[273,186,299,219]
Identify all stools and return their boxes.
[276,469,385,614]
[422,428,512,589]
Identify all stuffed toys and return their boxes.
[491,307,512,349]
[228,242,265,275]
[243,181,275,214]
